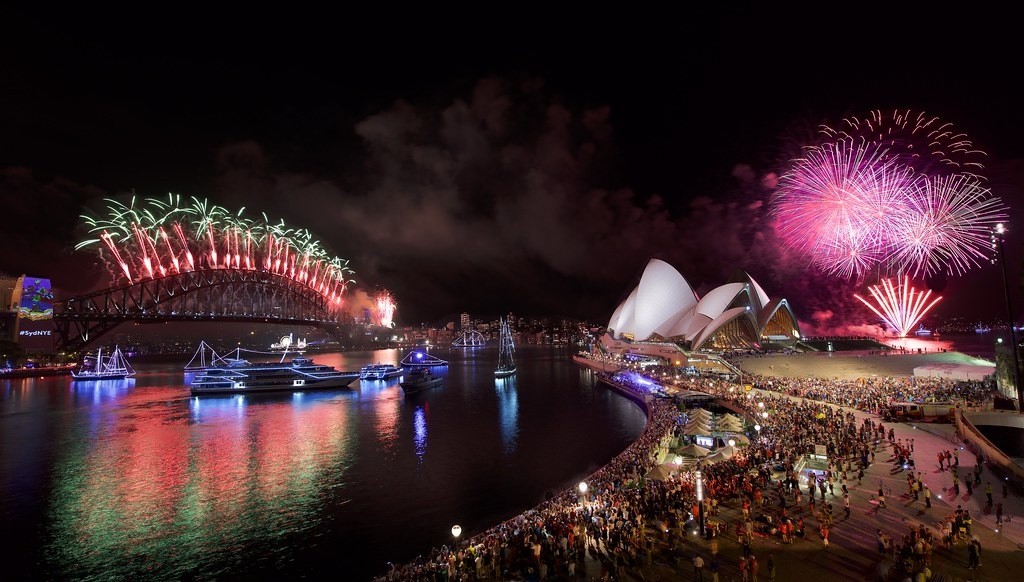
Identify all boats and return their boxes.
[400,369,444,394]
[401,351,448,366]
[360,362,403,380]
[190,332,360,394]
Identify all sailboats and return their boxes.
[493,316,517,377]
[451,330,486,347]
[70,344,136,379]
[184,339,228,370]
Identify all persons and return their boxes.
[373,335,1024,582]
